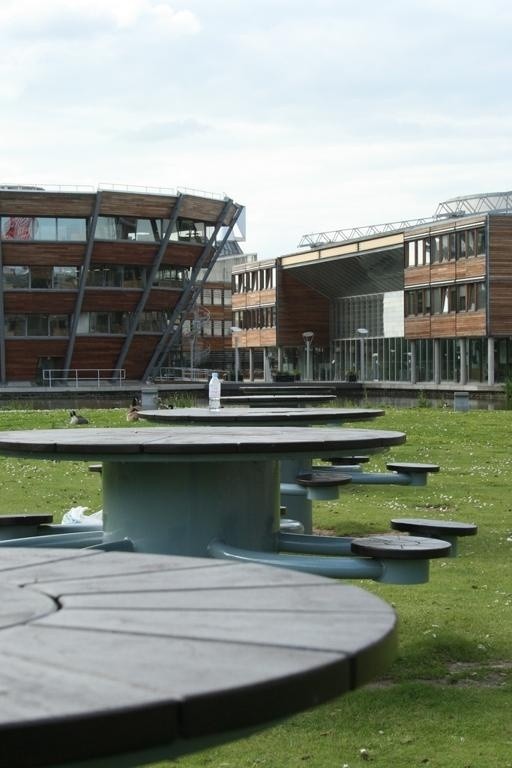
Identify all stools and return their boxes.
[346,463,440,487]
[347,515,477,585]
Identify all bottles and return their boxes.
[209,372,221,410]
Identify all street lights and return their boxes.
[355,328,368,383]
[230,326,243,381]
[302,331,314,381]
